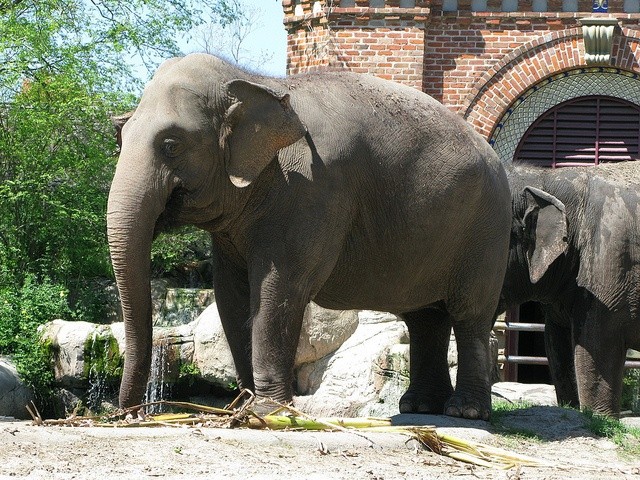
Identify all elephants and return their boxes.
[108,51,511,421]
[502,162,640,424]
